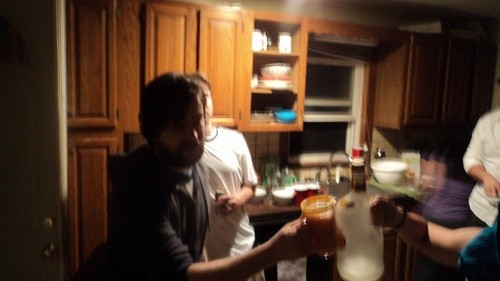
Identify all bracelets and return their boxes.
[393,209,408,229]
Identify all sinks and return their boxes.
[318,183,390,203]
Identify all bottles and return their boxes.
[335,146,384,281]
[361,144,369,181]
[277,31,291,53]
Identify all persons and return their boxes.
[369,104,500,281]
[109,73,348,281]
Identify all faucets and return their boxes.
[306,149,352,186]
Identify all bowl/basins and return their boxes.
[271,190,294,205]
[248,188,267,204]
[370,159,409,186]
[274,108,296,123]
[258,62,292,88]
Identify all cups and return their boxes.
[300,194,335,256]
[251,27,266,51]
[294,182,320,208]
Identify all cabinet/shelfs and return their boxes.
[66,0,499,281]
